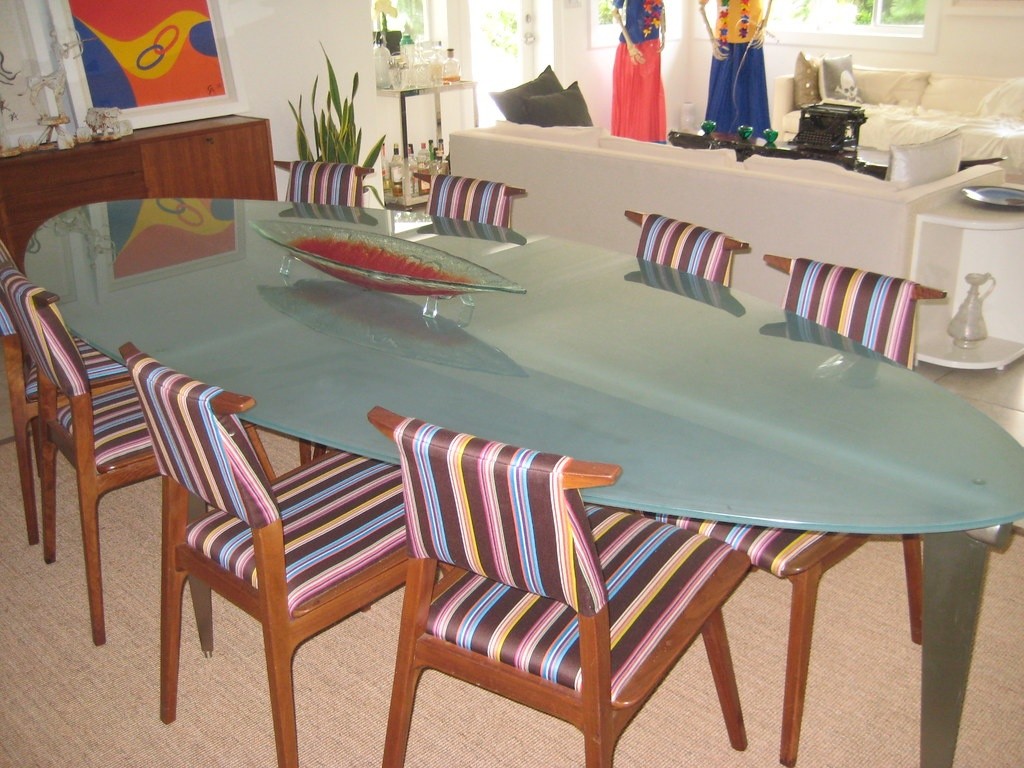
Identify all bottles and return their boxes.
[379,138,452,197]
[373,33,462,90]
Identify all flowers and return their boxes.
[375,0,397,28]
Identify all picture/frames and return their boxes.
[49,1,242,134]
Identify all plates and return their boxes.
[960,184,1024,212]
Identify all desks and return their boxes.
[22,199,1024,768]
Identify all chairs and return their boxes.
[654,254,938,766]
[415,171,526,229]
[0,270,169,646]
[368,406,752,768]
[625,208,744,289]
[119,342,412,767]
[272,154,375,208]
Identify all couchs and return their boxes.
[772,68,1024,172]
[448,121,1005,291]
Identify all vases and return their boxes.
[373,30,402,56]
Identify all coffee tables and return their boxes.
[669,128,888,179]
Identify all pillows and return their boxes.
[488,66,563,124]
[526,81,595,128]
[818,54,862,105]
[793,53,820,105]
[887,125,966,183]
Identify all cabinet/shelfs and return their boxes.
[0,136,144,264]
[136,110,277,202]
[378,81,479,210]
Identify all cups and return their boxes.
[701,120,716,133]
[737,125,754,138]
[762,128,778,144]
